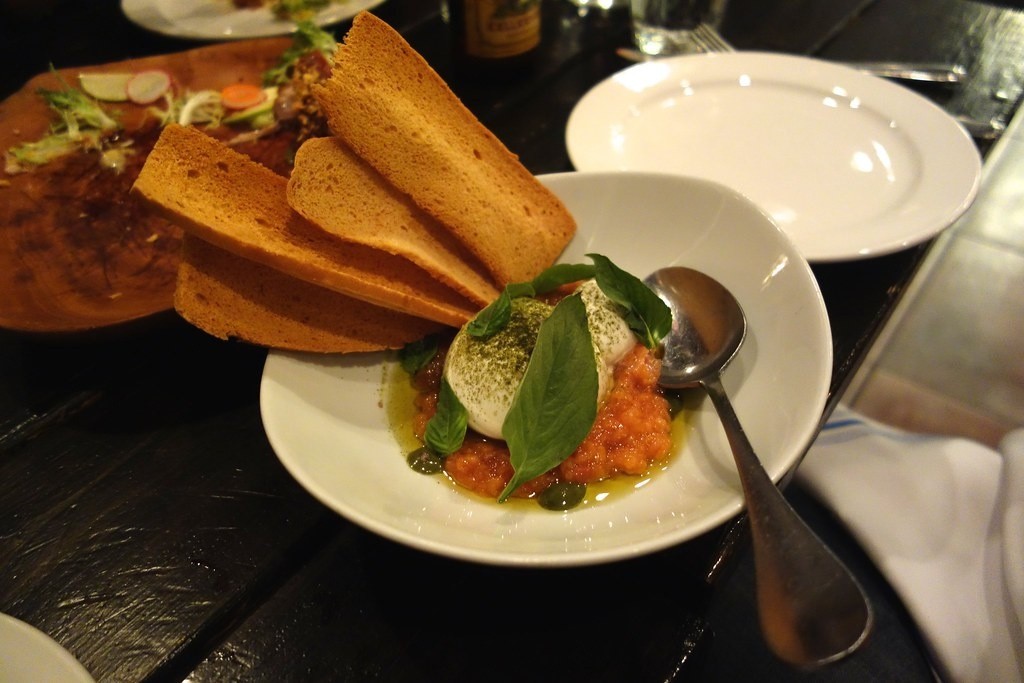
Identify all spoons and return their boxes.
[642,267,874,670]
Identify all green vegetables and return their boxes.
[9,0,346,170]
[400,253,671,501]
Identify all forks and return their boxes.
[685,23,1003,141]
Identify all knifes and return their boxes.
[616,44,965,85]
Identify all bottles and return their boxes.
[440,0,545,72]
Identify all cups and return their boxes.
[630,0,725,53]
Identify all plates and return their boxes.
[0,612,98,683]
[120,0,385,41]
[2,40,310,331]
[260,172,834,569]
[565,52,981,262]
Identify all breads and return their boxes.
[130,11,576,353]
